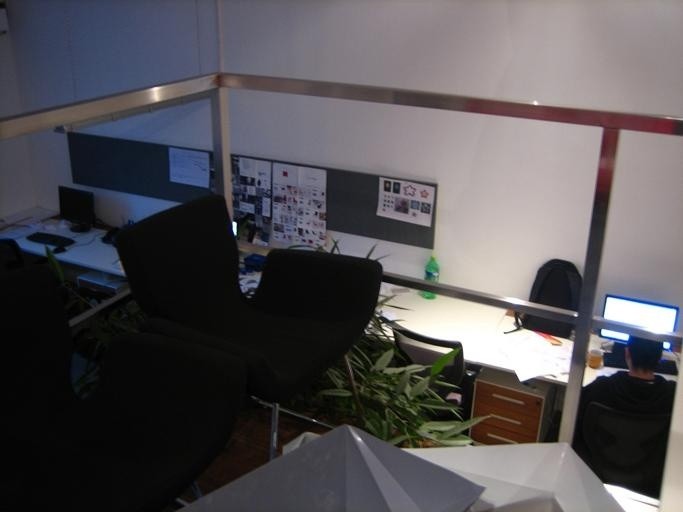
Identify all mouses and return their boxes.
[53,247,66,254]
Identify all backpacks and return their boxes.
[519,258,583,338]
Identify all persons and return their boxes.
[571,334,676,501]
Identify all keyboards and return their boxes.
[26,232,75,247]
[603,351,678,375]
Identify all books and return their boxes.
[553,346,572,375]
[508,328,559,382]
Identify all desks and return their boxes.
[0,207,127,274]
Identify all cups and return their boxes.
[588,349,602,369]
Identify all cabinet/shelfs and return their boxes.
[76,273,126,297]
[468,367,555,446]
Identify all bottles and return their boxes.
[420,254,440,299]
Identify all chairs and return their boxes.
[390,319,478,420]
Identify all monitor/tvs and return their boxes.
[598,294,679,353]
[58,185,94,233]
